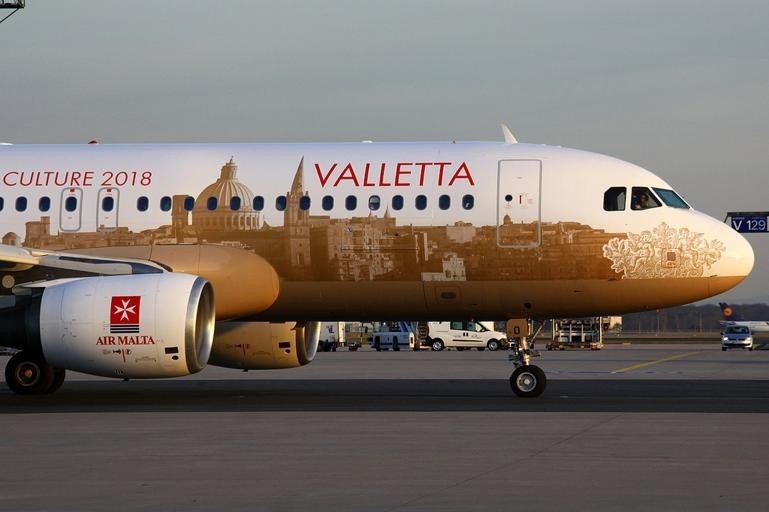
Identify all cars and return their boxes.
[720,325,753,352]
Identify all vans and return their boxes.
[427,320,509,350]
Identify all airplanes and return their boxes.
[0,123,756,398]
[716,297,768,335]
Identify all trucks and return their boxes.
[316,319,360,352]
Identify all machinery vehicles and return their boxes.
[372,316,414,352]
